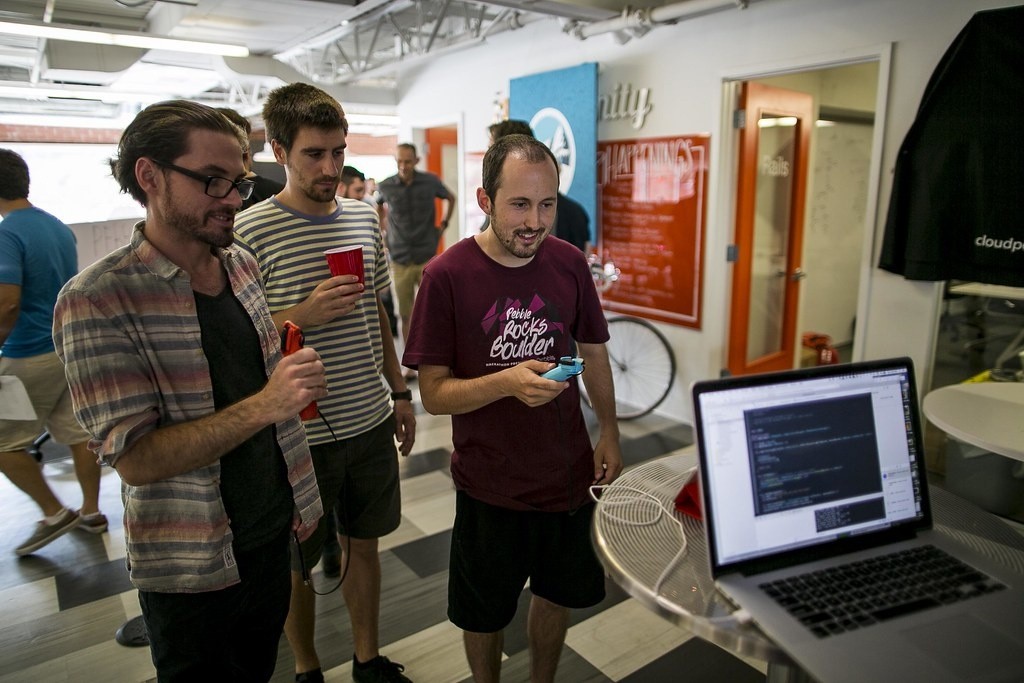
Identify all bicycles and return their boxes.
[570,252,678,421]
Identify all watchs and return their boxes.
[389,388,414,403]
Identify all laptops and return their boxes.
[691,357,1024,683]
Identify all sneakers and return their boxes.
[14,510,81,554]
[76,508,108,532]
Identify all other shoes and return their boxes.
[353,650,412,683]
[402,368,417,380]
[294,667,326,683]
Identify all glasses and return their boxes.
[148,157,255,200]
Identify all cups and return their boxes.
[324,245,365,296]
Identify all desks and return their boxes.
[923,382,1024,462]
[591,444,1024,683]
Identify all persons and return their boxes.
[218,108,283,211]
[50,102,331,683]
[376,142,456,383]
[402,136,624,683]
[336,164,401,338]
[477,118,591,253]
[230,83,416,683]
[0,145,110,560]
